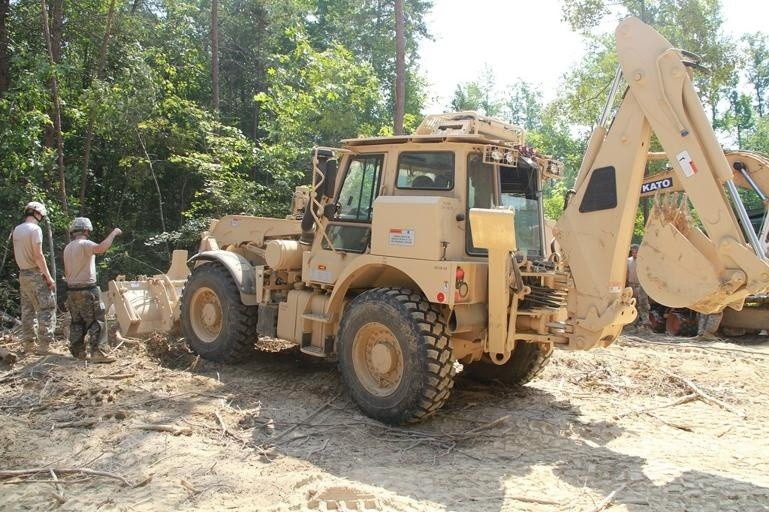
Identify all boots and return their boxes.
[77,351,115,363]
[24,342,63,356]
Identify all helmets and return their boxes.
[69,217,93,232]
[24,202,46,217]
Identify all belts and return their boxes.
[67,285,96,290]
[20,267,38,272]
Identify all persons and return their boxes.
[12,202,58,355]
[63,217,123,364]
[697,312,722,340]
[626,244,650,332]
